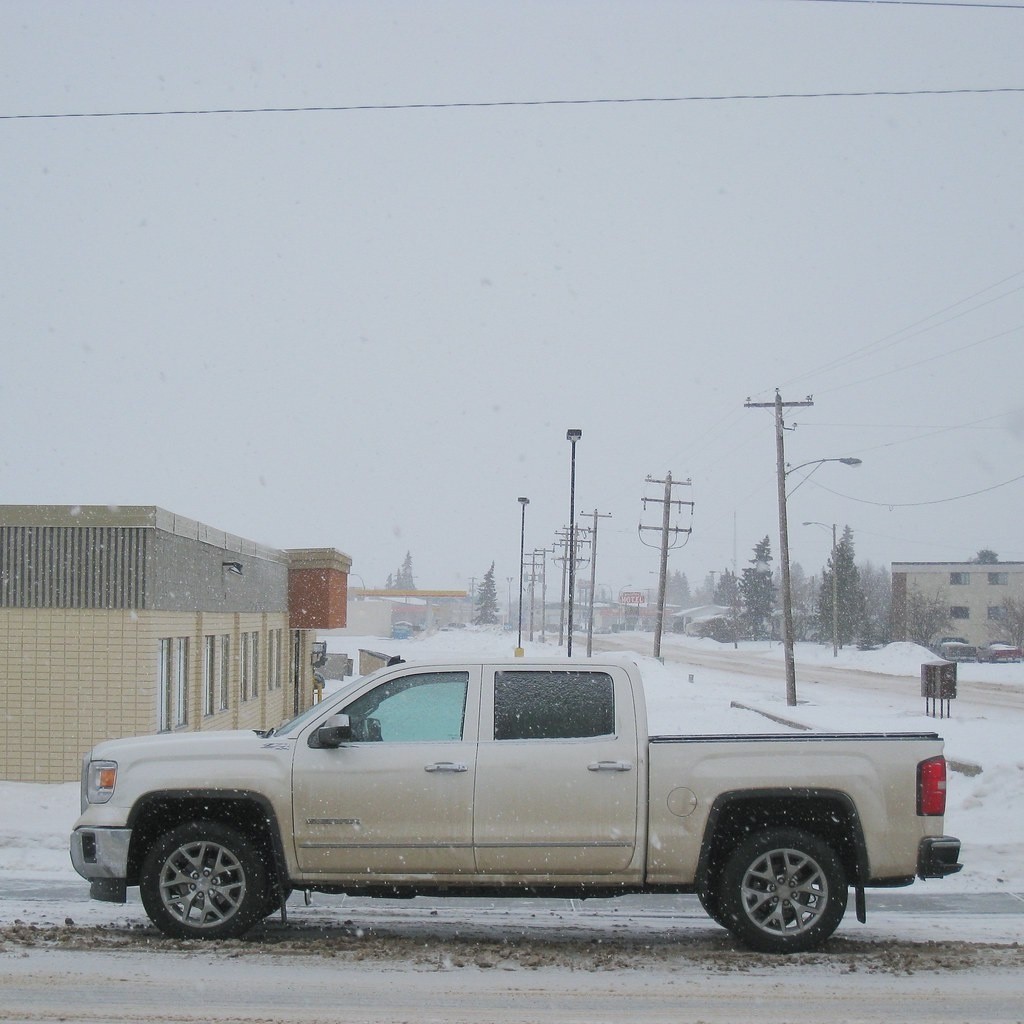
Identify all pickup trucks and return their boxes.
[940,636,978,663]
[977,640,1019,664]
[64,654,965,957]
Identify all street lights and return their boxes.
[708,569,759,642]
[506,577,513,629]
[600,571,668,635]
[800,518,841,658]
[778,457,864,706]
[566,428,585,658]
[516,496,531,657]
